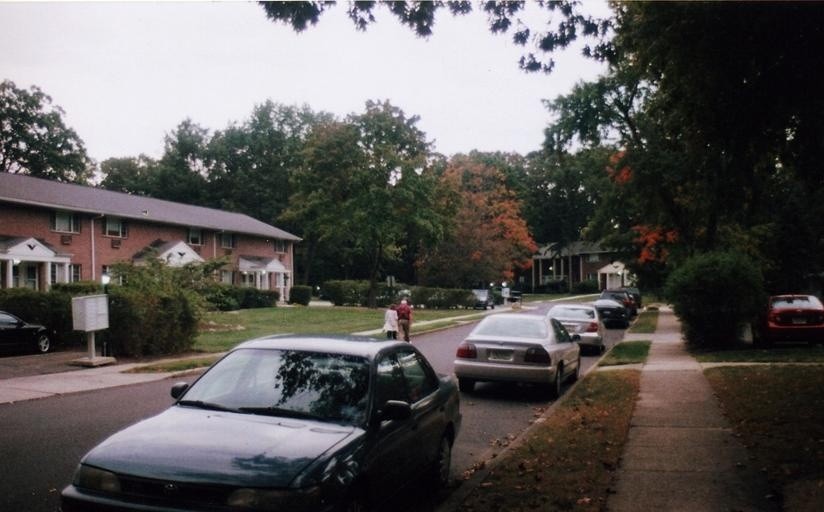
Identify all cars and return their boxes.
[763,295,824,348]
[544,284,642,354]
[453,311,583,396]
[465,288,495,310]
[54,333,462,509]
[0,309,51,356]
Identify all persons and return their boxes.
[397,299,413,343]
[383,304,399,341]
